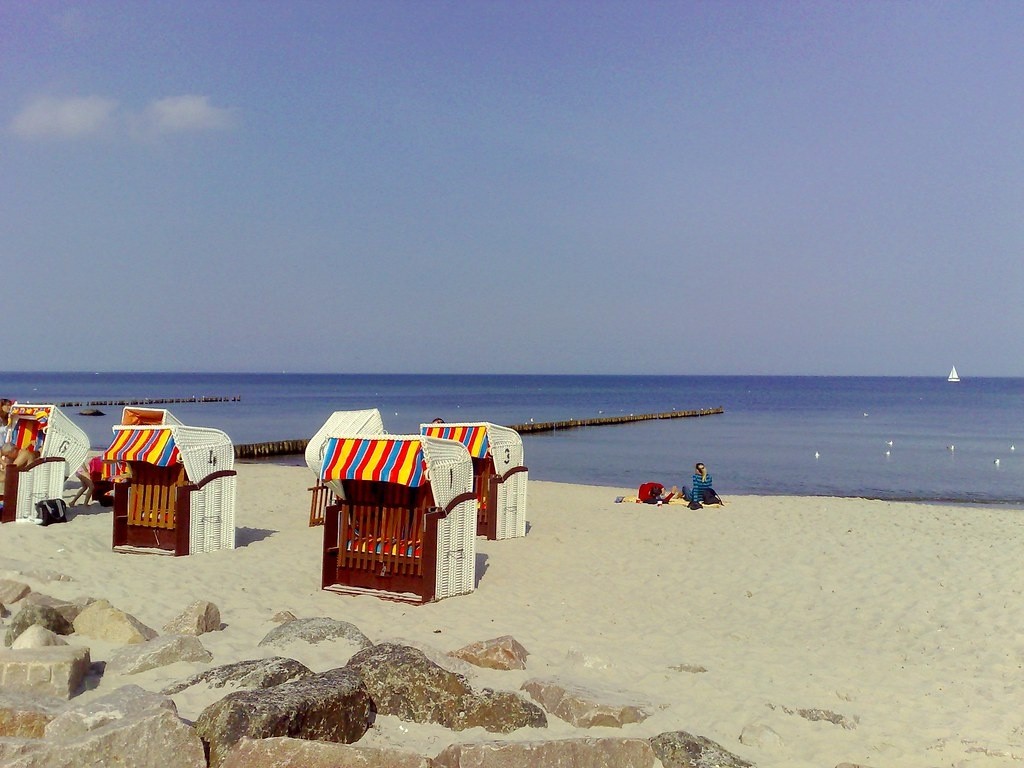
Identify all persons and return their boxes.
[638,482,677,504]
[0,443,36,482]
[0,398,12,442]
[68,456,99,507]
[673,463,712,505]
[432,417,443,423]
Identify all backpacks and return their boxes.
[702,488,724,506]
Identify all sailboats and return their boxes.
[947,365,960,382]
